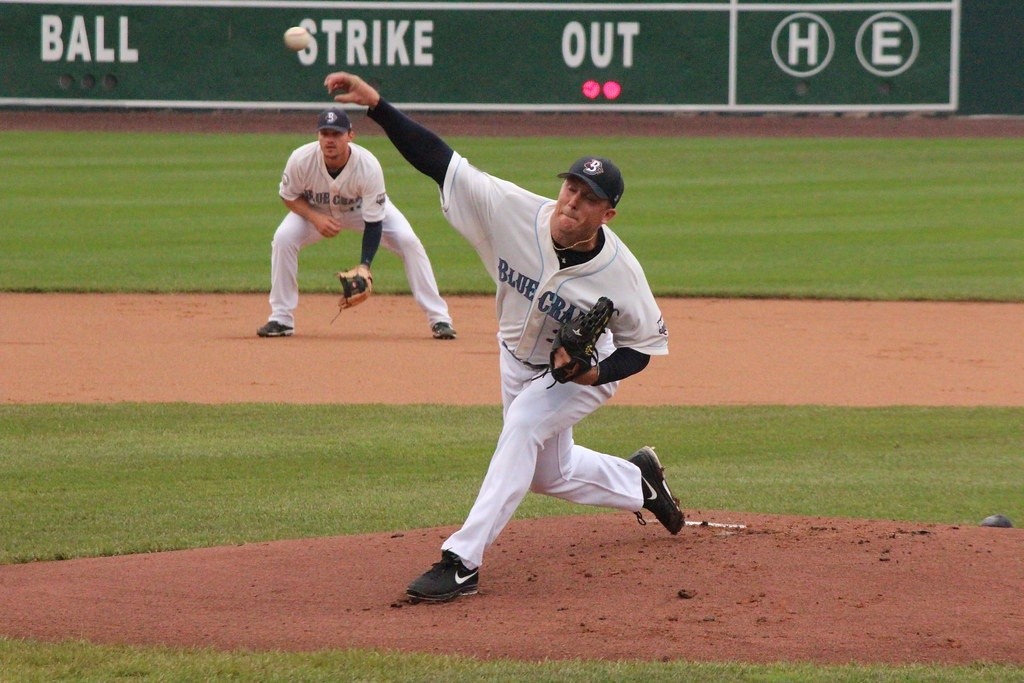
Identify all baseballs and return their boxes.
[284,26,311,54]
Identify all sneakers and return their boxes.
[406,547,480,604]
[626,445,685,536]
[431,322,457,340]
[257,321,295,337]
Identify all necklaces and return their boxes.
[552,231,598,250]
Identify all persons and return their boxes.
[255,108,456,339]
[323,73,684,601]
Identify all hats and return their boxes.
[316,107,353,134]
[557,155,624,210]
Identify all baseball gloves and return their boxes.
[327,264,375,324]
[533,295,622,392]
